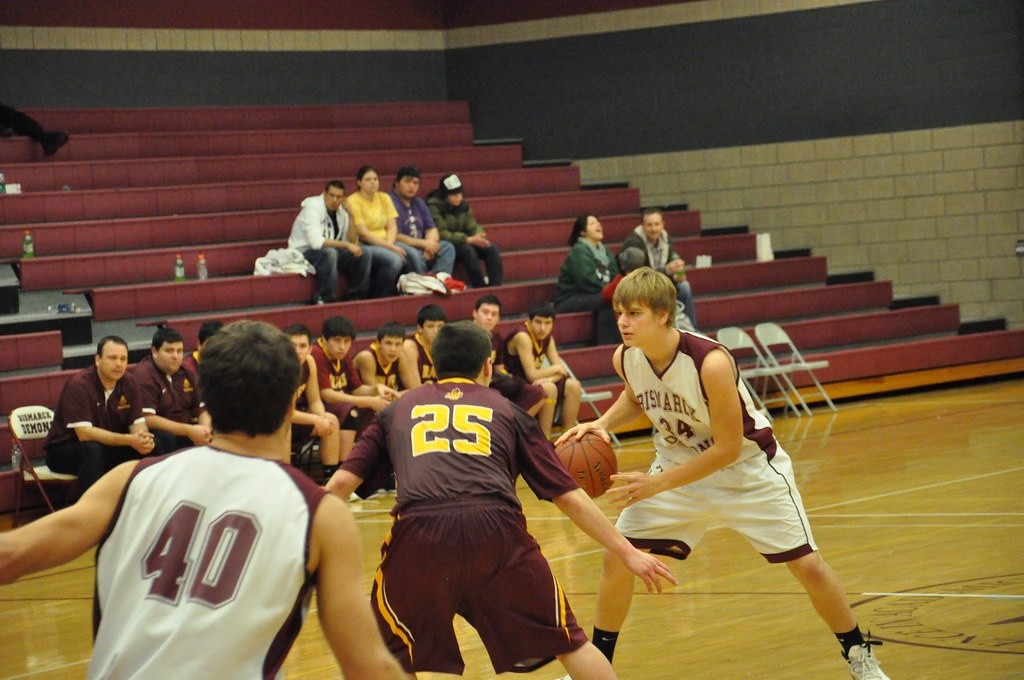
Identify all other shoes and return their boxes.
[347,492,360,502]
[42,129,69,157]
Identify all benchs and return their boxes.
[0,102,1023,531]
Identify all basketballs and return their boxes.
[554,432,618,500]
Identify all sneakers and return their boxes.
[841,630,890,680]
[556,673,571,680]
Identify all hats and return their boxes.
[440,175,464,194]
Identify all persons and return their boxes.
[619,207,697,333]
[472,296,547,418]
[390,166,456,275]
[403,303,445,385]
[45,335,156,493]
[283,324,359,503]
[134,328,213,458]
[182,320,224,383]
[287,180,371,303]
[310,317,400,497]
[321,319,680,680]
[555,266,892,680]
[0,321,410,680]
[0,104,68,157]
[425,174,503,288]
[343,166,426,299]
[601,247,645,324]
[504,301,582,441]
[352,320,418,390]
[554,214,624,346]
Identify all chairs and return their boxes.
[540,357,621,449]
[8,406,79,529]
[716,326,801,421]
[753,323,837,416]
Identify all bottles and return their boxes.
[11,438,23,472]
[48,302,81,313]
[197,253,208,280]
[174,254,186,283]
[23,230,34,259]
[670,249,685,281]
